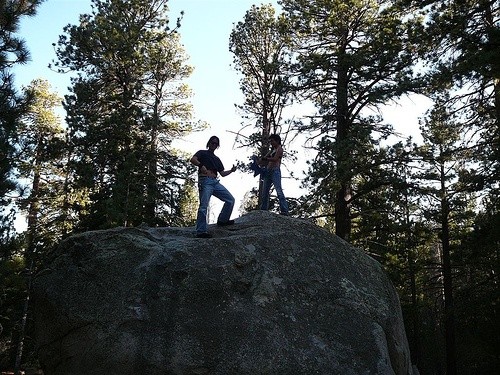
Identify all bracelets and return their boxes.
[199,163,203,168]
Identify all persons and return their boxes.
[257,134,289,216]
[192,136,237,238]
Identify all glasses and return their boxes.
[211,141,218,146]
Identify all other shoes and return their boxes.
[217,220,235,226]
[197,232,214,238]
